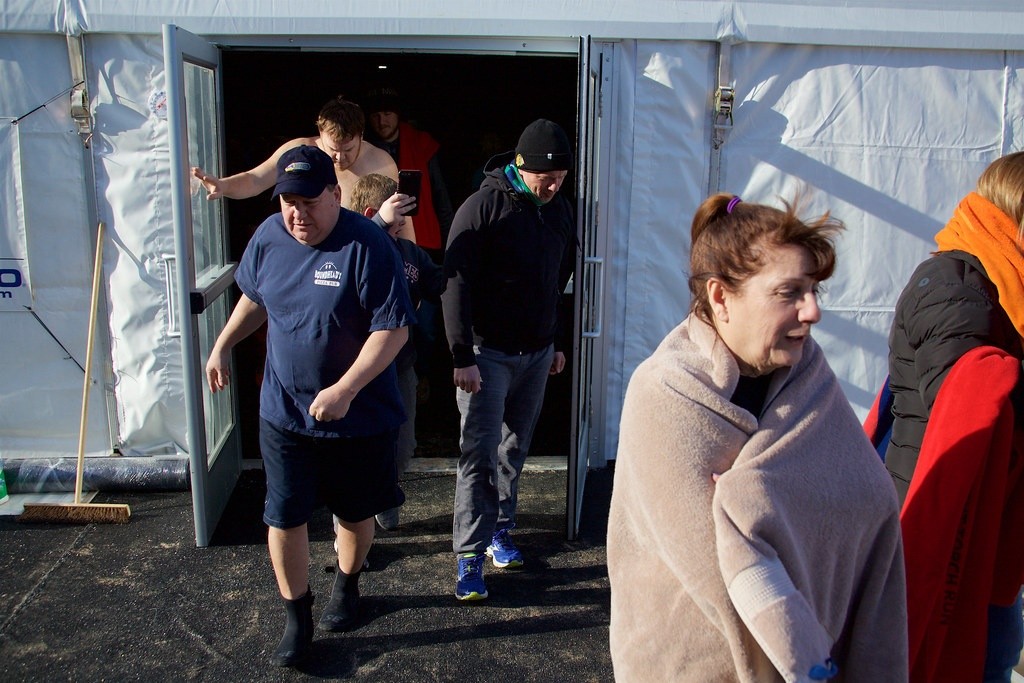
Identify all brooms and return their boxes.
[15,221,133,527]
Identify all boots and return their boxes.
[318,570,362,632]
[270,587,313,669]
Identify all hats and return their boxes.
[515,118,572,171]
[271,143,337,200]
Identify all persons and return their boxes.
[438,117,578,602]
[604,187,912,683]
[879,150,1023,680]
[204,144,422,668]
[350,85,461,291]
[330,172,443,580]
[191,94,416,255]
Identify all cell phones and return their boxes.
[397,169,422,216]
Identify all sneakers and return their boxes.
[453,549,489,600]
[484,531,523,567]
[377,507,399,530]
